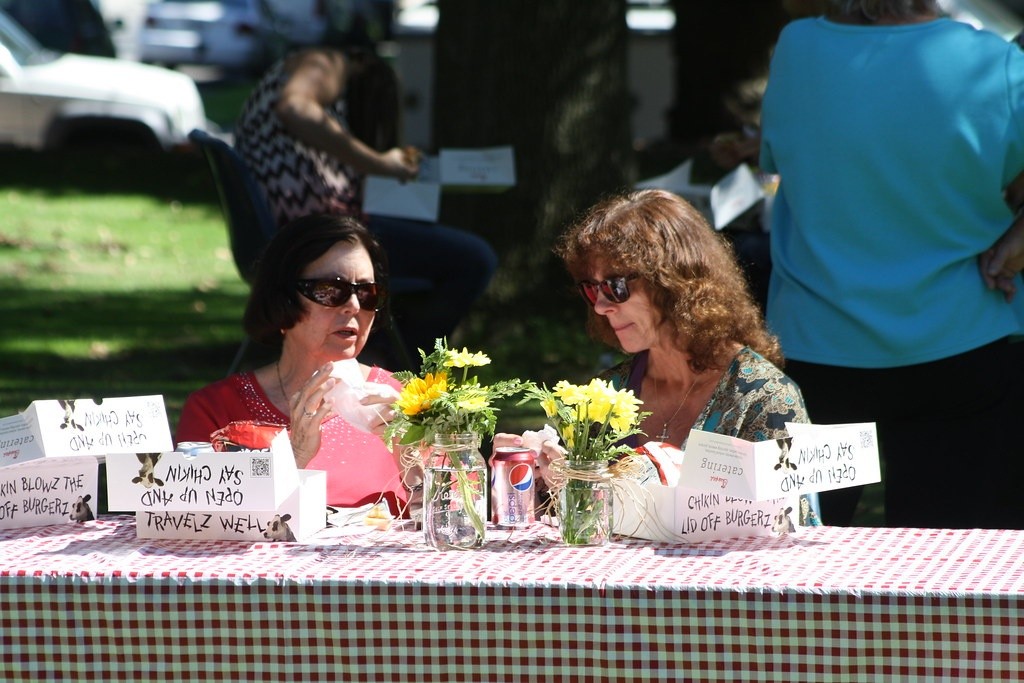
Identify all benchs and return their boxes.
[0,513,1024,683]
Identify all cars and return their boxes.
[134,0,288,80]
[0,14,210,184]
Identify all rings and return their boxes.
[303,407,312,418]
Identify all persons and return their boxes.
[175,213,477,530]
[562,188,825,528]
[230,32,498,368]
[757,0,1024,528]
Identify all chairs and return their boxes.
[187,129,439,379]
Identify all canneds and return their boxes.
[175,440,215,456]
[490,446,536,529]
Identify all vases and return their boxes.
[560,462,614,545]
[423,434,488,552]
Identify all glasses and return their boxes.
[575,271,649,306]
[285,276,380,310]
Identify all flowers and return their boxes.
[517,378,654,546]
[381,336,538,552]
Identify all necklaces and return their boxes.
[652,368,701,443]
[274,360,290,405]
